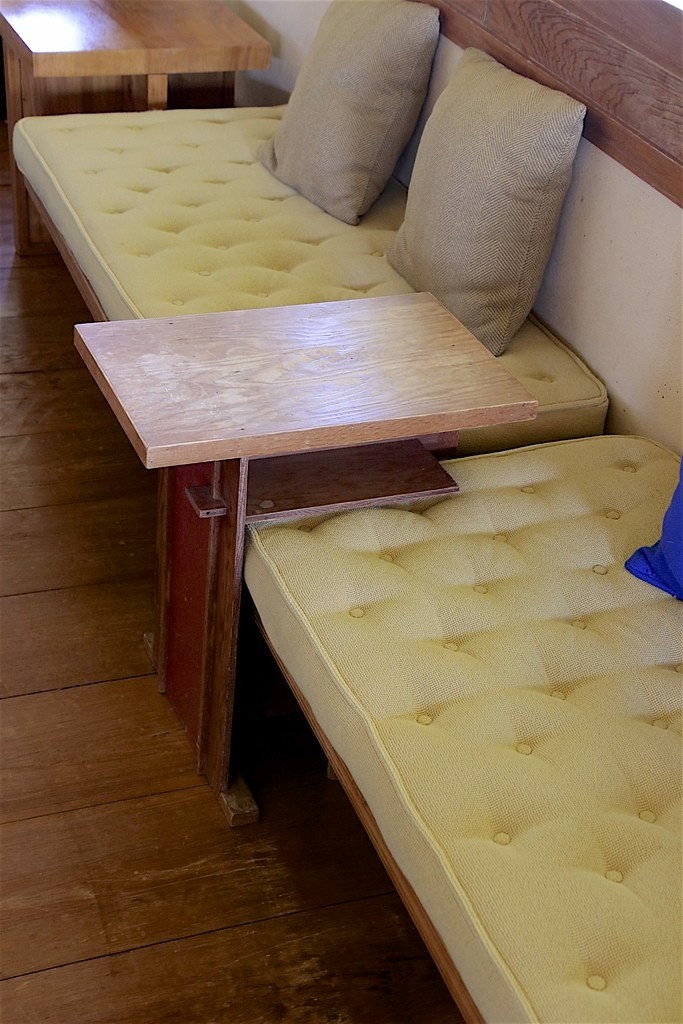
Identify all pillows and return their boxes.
[257,0,441,223]
[383,42,586,353]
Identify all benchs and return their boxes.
[15,42,683,1023]
[0,1,272,172]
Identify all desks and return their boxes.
[76,291,538,829]
